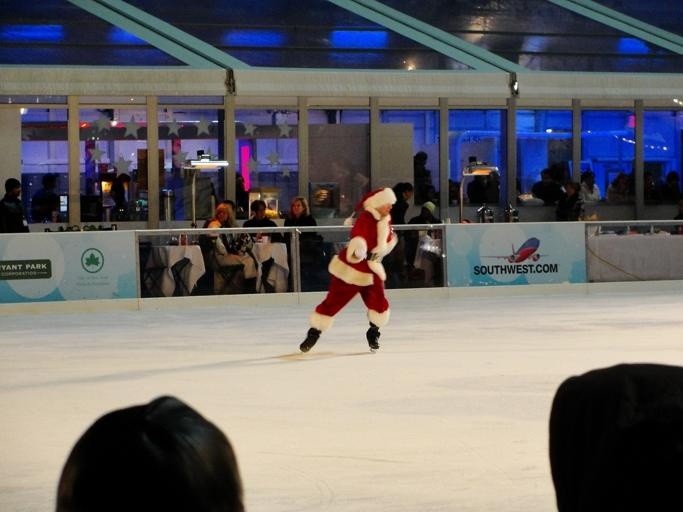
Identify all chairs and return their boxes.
[199,235,250,294]
[138,242,167,297]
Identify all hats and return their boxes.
[421,201,436,214]
[353,186,397,208]
[6,179,23,192]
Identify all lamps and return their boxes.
[180,149,229,244]
[458,155,499,224]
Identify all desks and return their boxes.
[251,241,290,293]
[153,243,206,297]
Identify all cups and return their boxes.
[261,233,269,243]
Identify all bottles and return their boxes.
[178,232,193,245]
[110,224,117,230]
[650,223,655,234]
[624,224,631,236]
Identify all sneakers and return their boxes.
[366,326,380,349]
[300,328,321,352]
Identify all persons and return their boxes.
[184,168,214,220]
[414,152,461,205]
[548,363,683,512]
[199,197,331,294]
[1,178,29,233]
[532,164,601,221]
[311,189,330,208]
[235,174,249,220]
[31,172,60,223]
[606,174,681,204]
[467,171,500,204]
[95,174,131,221]
[300,186,399,353]
[382,182,444,289]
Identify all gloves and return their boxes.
[354,248,366,259]
